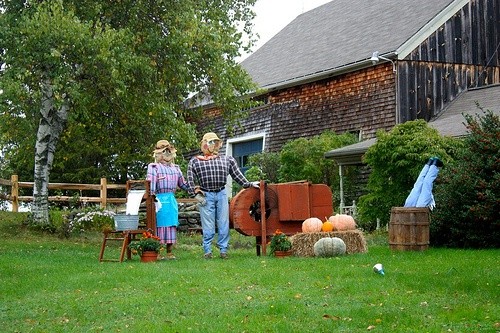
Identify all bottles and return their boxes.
[373,263,385,276]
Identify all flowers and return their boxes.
[269,229,292,256]
[128,231,167,256]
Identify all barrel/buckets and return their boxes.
[388,206,430,252]
[112,213,139,232]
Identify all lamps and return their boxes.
[369,52,395,73]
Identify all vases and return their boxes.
[274,250,293,258]
[141,251,158,262]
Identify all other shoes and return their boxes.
[166,253,176,260]
[220,253,229,259]
[157,254,162,261]
[205,253,213,260]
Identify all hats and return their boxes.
[202,132,221,142]
[152,140,177,154]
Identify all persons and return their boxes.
[404,156,445,207]
[147,139,206,260]
[187,132,260,259]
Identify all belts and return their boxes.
[200,186,225,192]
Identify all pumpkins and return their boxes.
[302,214,356,233]
[313,233,345,257]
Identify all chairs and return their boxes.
[100,180,151,262]
